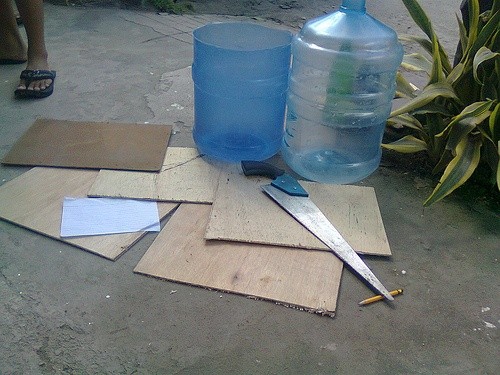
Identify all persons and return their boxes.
[0,0,56,98]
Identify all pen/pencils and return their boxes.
[358,288,403,305]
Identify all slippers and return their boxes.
[0,59,27,65]
[14,69,57,97]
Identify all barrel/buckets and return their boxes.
[191,21,294,163]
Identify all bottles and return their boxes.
[281,0,403,185]
